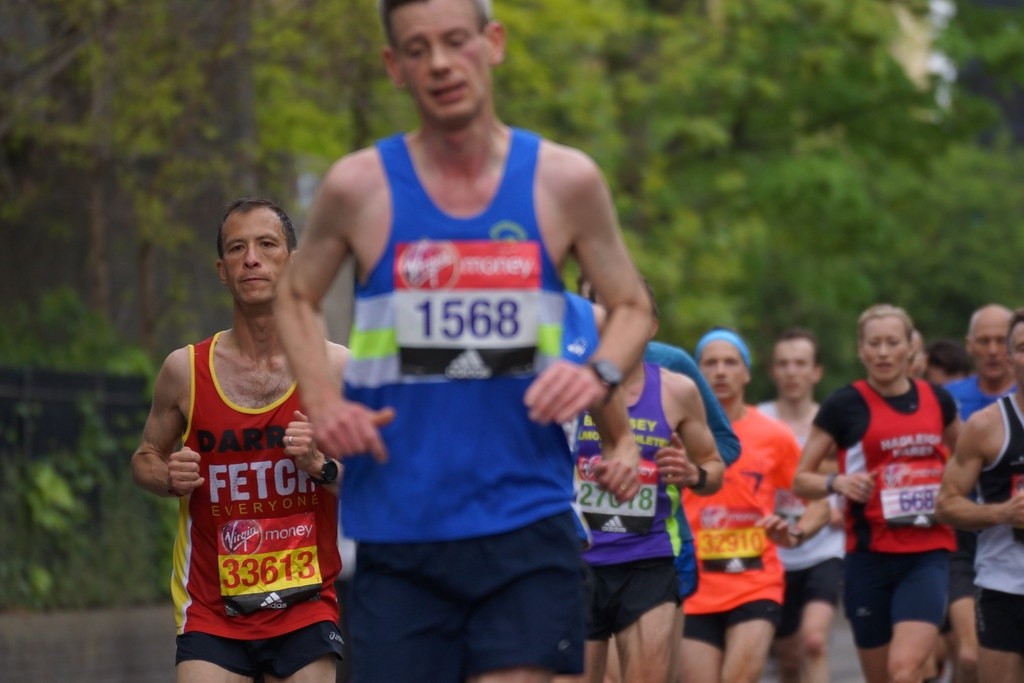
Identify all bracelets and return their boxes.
[826,472,843,497]
[690,465,707,490]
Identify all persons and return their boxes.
[577,266,742,683]
[132,199,351,683]
[792,303,966,683]
[817,328,974,683]
[752,332,848,683]
[936,308,1024,683]
[940,303,1018,683]
[555,284,725,683]
[678,330,832,683]
[268,0,662,683]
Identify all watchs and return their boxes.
[309,454,338,486]
[788,527,804,548]
[585,359,624,405]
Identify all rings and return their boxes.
[619,485,626,491]
[288,436,293,445]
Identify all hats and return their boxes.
[694,328,751,369]
